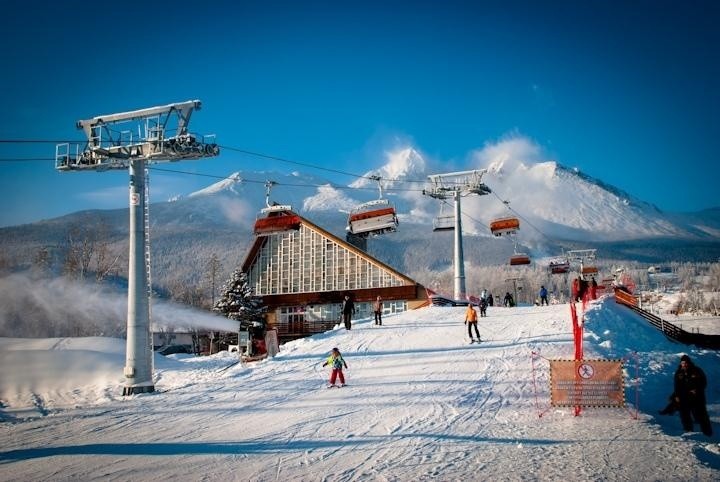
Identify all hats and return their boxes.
[681,355,690,361]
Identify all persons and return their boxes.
[323,348,347,387]
[342,296,355,330]
[374,296,383,325]
[465,304,481,343]
[572,277,597,303]
[504,292,513,307]
[675,355,712,439]
[540,285,548,306]
[480,290,493,317]
